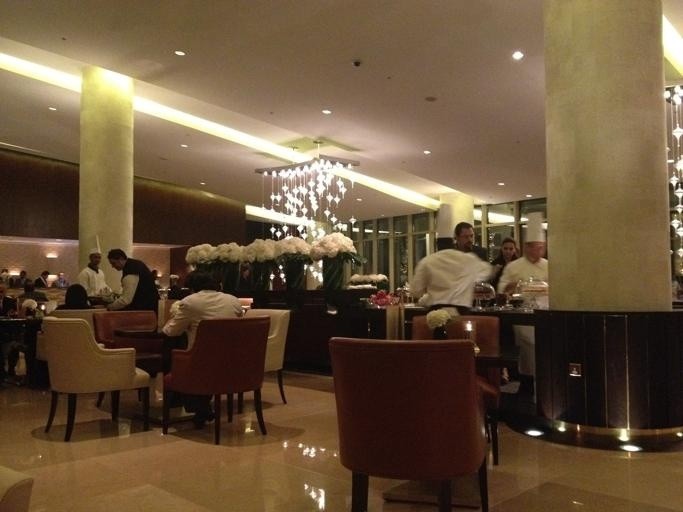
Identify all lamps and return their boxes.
[662,77,682,272]
[254,137,365,238]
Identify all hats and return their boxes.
[525,212,547,243]
[88,234,103,254]
[437,203,456,239]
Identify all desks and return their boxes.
[112,324,212,427]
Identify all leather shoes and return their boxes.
[192,408,213,423]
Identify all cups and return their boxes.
[15,298,26,318]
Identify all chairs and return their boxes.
[409,313,510,467]
[329,335,494,511]
[1,277,170,390]
[160,316,272,444]
[92,309,158,410]
[41,316,152,443]
[242,306,291,410]
[157,298,182,330]
[238,297,253,316]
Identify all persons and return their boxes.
[152,270,157,282]
[104,248,160,320]
[410,203,500,339]
[0,269,49,319]
[76,234,111,299]
[51,272,72,288]
[498,212,548,392]
[55,284,95,309]
[491,237,520,292]
[453,221,489,262]
[161,270,243,426]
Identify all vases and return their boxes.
[284,262,306,290]
[432,327,445,340]
[197,262,215,287]
[322,257,348,293]
[219,262,240,290]
[250,262,270,294]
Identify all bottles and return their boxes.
[59,273,63,288]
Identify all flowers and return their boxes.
[240,237,277,268]
[310,232,362,266]
[208,242,240,266]
[272,238,315,265]
[185,242,215,265]
[426,309,449,330]
